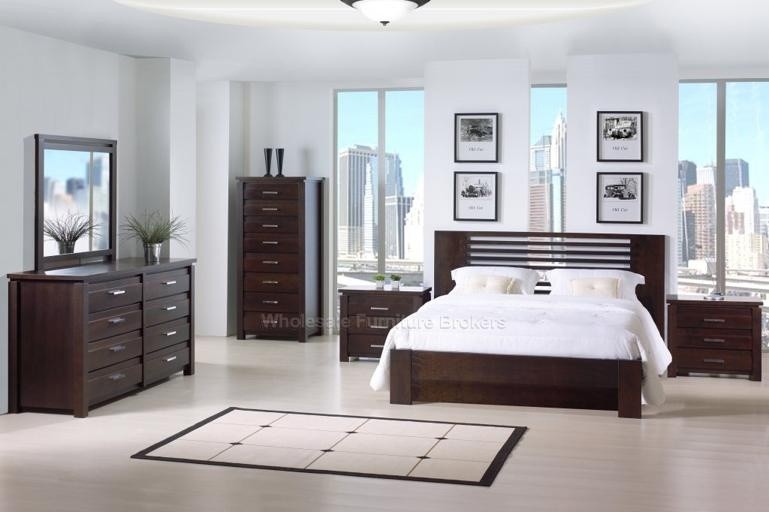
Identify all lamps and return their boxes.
[341,0,430,29]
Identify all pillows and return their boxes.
[449,266,646,303]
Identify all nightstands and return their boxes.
[337,286,432,362]
[666,295,763,381]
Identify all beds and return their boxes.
[389,230,665,419]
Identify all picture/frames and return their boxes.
[454,170,498,222]
[596,171,643,224]
[454,111,498,164]
[596,111,644,163]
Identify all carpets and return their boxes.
[130,407,527,487]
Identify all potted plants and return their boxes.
[391,275,401,289]
[375,275,385,288]
[42,210,105,255]
[119,208,193,262]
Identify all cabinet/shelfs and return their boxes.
[235,177,324,343]
[6,257,197,418]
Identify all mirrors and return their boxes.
[34,133,117,274]
